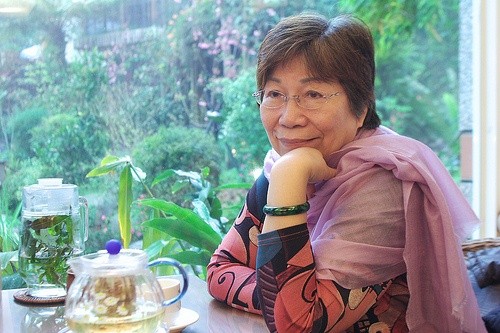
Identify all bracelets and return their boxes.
[262,201,311,217]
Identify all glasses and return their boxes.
[252,89,346,109]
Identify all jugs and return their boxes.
[69,240,189,333]
[18,177,89,298]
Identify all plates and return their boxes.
[168,308,199,333]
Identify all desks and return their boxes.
[0,274,271,333]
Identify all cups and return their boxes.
[157,279,182,314]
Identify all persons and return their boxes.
[205,8,493,333]
